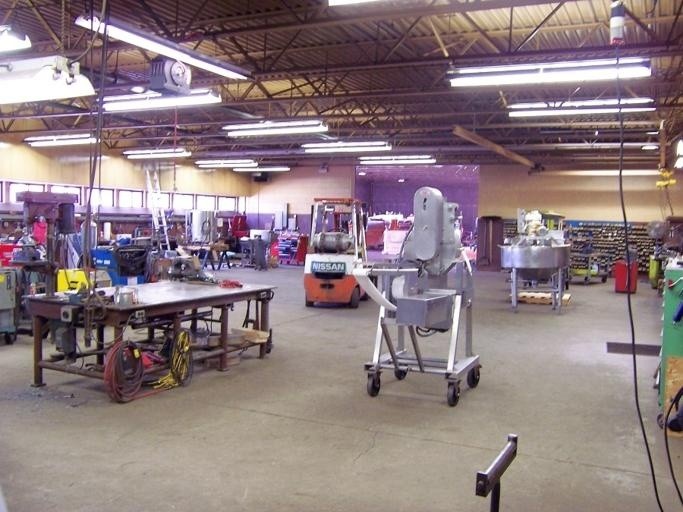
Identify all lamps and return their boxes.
[446,58,652,87]
[509,99,659,119]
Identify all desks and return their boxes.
[22,278,278,386]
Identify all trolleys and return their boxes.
[354,186,481,405]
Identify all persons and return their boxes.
[33,215,48,249]
[13,229,22,244]
